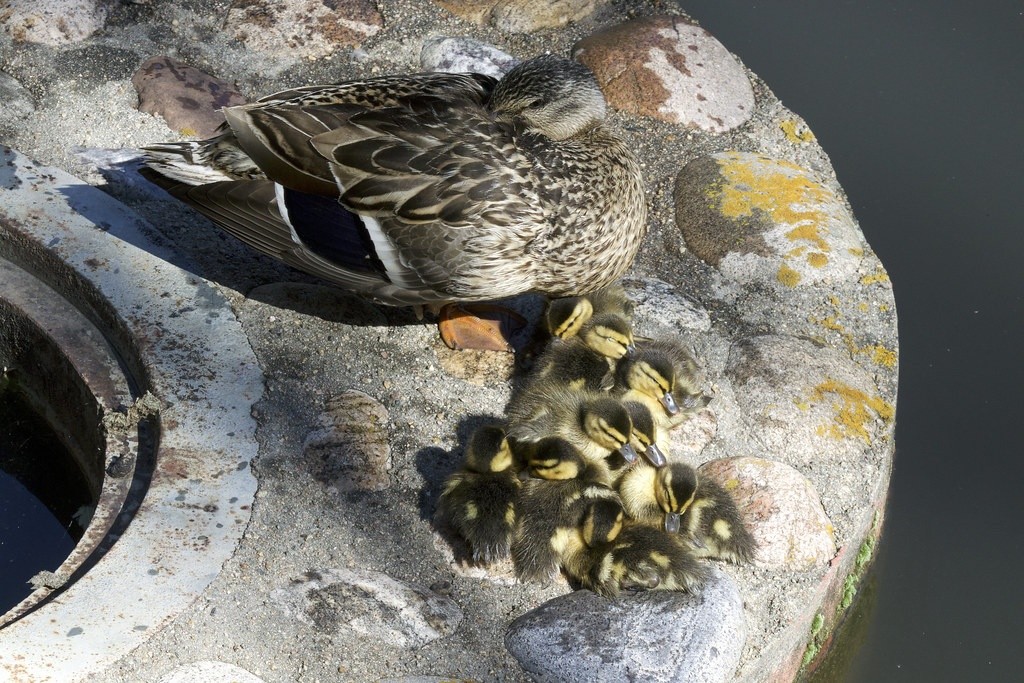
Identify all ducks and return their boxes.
[440,295,758,594]
[135,56,647,319]
